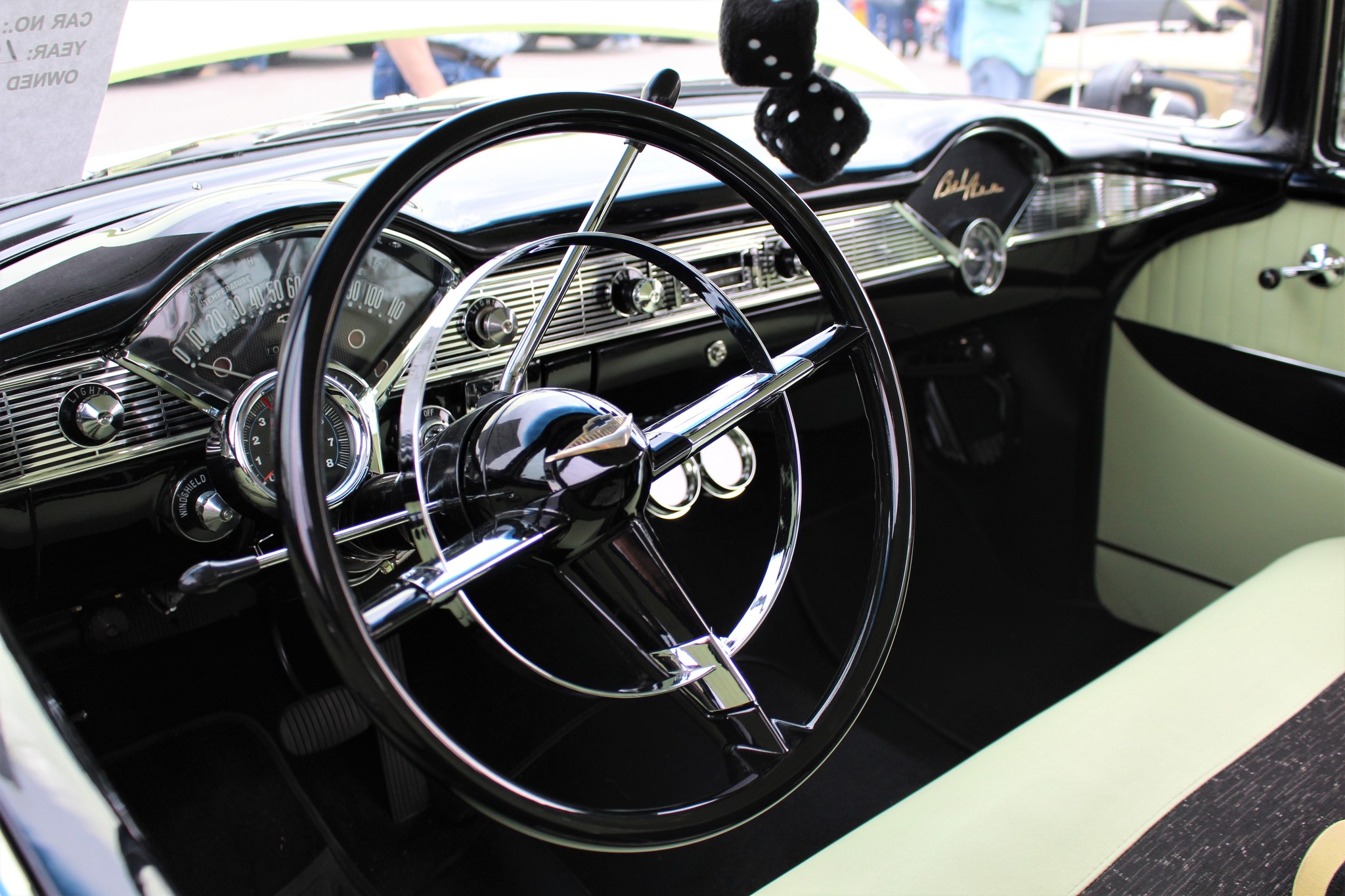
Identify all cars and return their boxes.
[1028,0,1266,123]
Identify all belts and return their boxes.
[428,41,499,75]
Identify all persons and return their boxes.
[865,1,1056,101]
[371,32,532,104]
